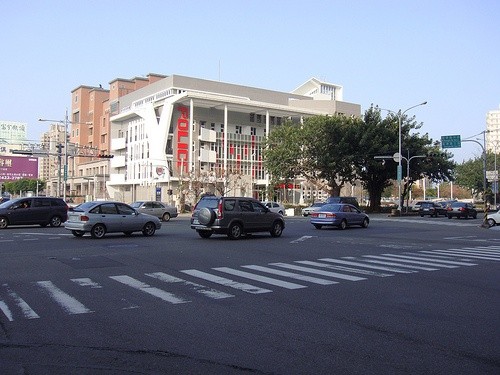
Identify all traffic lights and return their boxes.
[170,190,173,195]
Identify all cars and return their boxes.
[310,204,370,230]
[64,201,163,239]
[303,203,327,217]
[487,210,500,227]
[2,196,69,229]
[130,201,179,222]
[447,201,478,220]
[261,202,285,215]
[411,200,457,218]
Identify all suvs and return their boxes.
[190,196,285,240]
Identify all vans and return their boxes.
[324,197,359,208]
